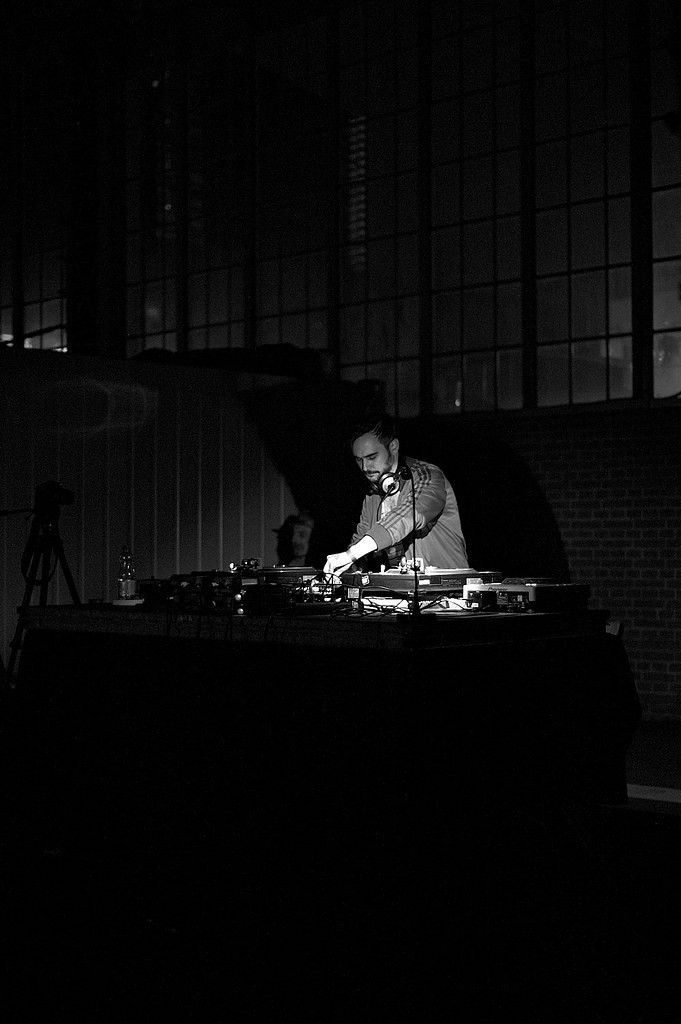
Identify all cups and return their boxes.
[88,588,108,604]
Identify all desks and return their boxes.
[22,602,636,814]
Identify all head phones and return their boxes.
[369,451,405,495]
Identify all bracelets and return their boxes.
[346,549,357,562]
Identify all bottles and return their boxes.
[117,545,136,601]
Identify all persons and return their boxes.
[318,417,470,586]
